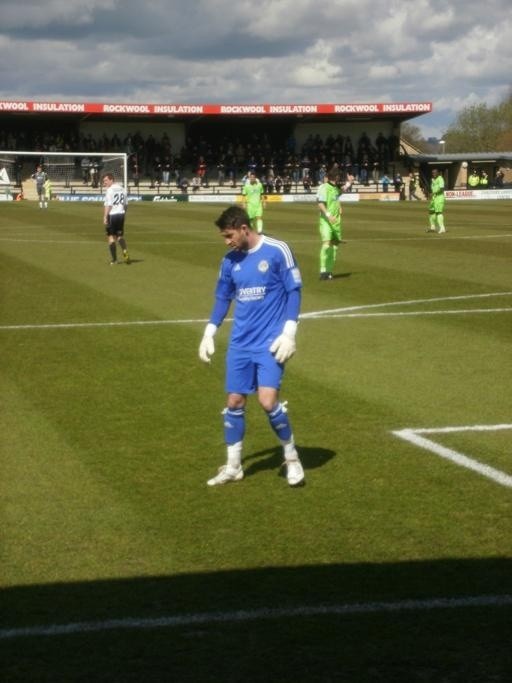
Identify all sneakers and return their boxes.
[277,457,306,486]
[205,464,244,487]
[111,252,130,265]
[319,271,334,281]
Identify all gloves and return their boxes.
[268,320,298,365]
[196,323,218,365]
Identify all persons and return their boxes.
[480,170,489,189]
[316,169,343,280]
[102,174,130,265]
[427,169,446,233]
[199,207,306,487]
[468,170,480,190]
[492,170,504,190]
[242,173,264,236]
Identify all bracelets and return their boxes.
[324,212,330,217]
